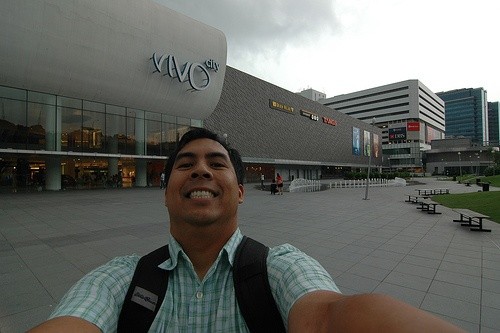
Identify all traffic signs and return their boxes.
[407,123,420,131]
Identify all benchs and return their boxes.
[451,209,492,232]
[405,188,449,214]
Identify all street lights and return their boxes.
[361,117,376,201]
[457,152,480,177]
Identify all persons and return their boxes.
[271,173,284,195]
[159,171,166,189]
[25,126,472,333]
[114,174,119,189]
[132,176,136,189]
[11,173,17,192]
[261,173,264,186]
